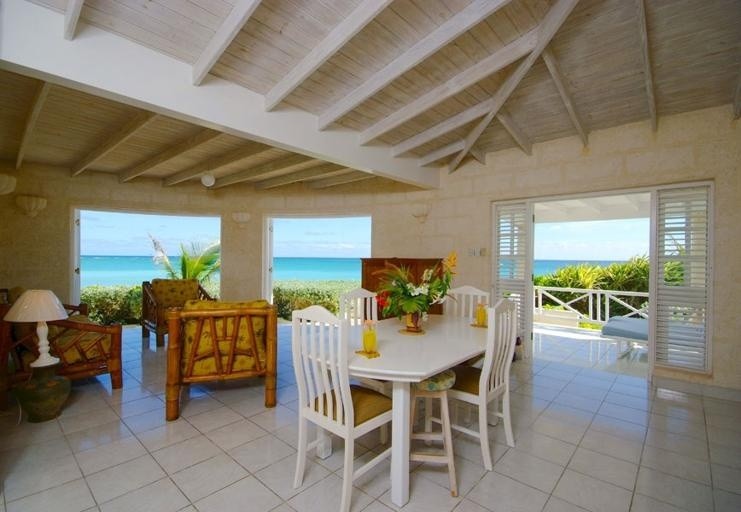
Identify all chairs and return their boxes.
[138,278,279,423]
[0,300,121,389]
[293,283,518,512]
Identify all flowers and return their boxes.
[371,250,465,315]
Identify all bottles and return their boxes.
[475,303,488,327]
[362,318,377,356]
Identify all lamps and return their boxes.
[200,173,216,188]
[0,173,47,218]
[2,290,70,366]
